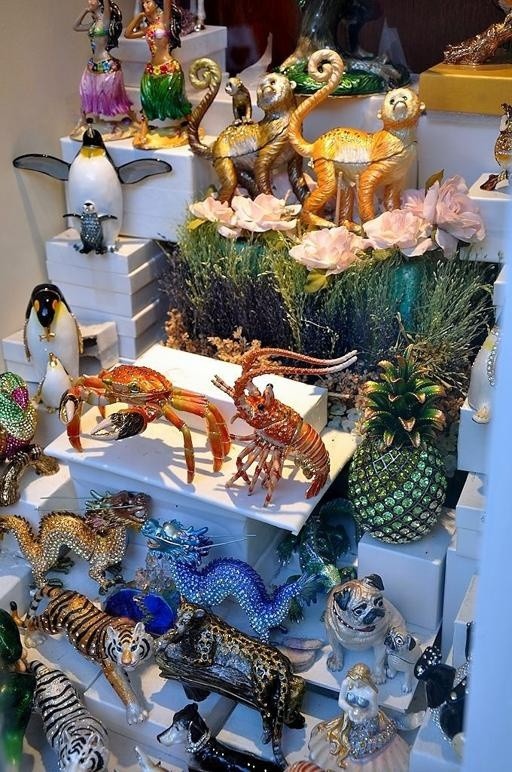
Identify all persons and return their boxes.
[72,1,136,127]
[124,1,191,121]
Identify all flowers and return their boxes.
[187,176,485,295]
